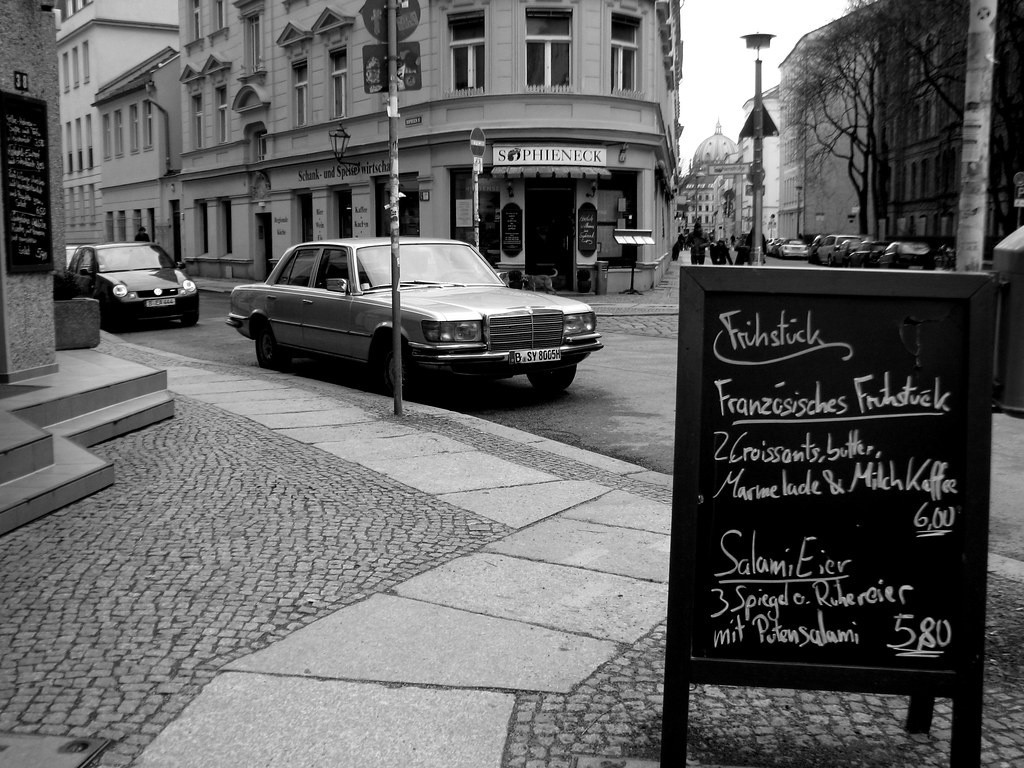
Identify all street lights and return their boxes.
[795,185,802,238]
[740,30,775,265]
[746,204,751,232]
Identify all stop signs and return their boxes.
[469,127,486,156]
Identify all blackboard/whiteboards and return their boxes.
[664,264,1004,696]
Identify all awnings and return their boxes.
[491,166,612,179]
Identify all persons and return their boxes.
[746,229,767,265]
[711,238,732,265]
[708,229,715,250]
[730,234,736,246]
[686,222,710,265]
[135,227,150,242]
[678,233,688,251]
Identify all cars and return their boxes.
[67,242,200,334]
[767,234,936,270]
[226,236,604,402]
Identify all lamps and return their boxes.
[328,122,361,176]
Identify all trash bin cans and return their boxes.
[595,261,608,296]
